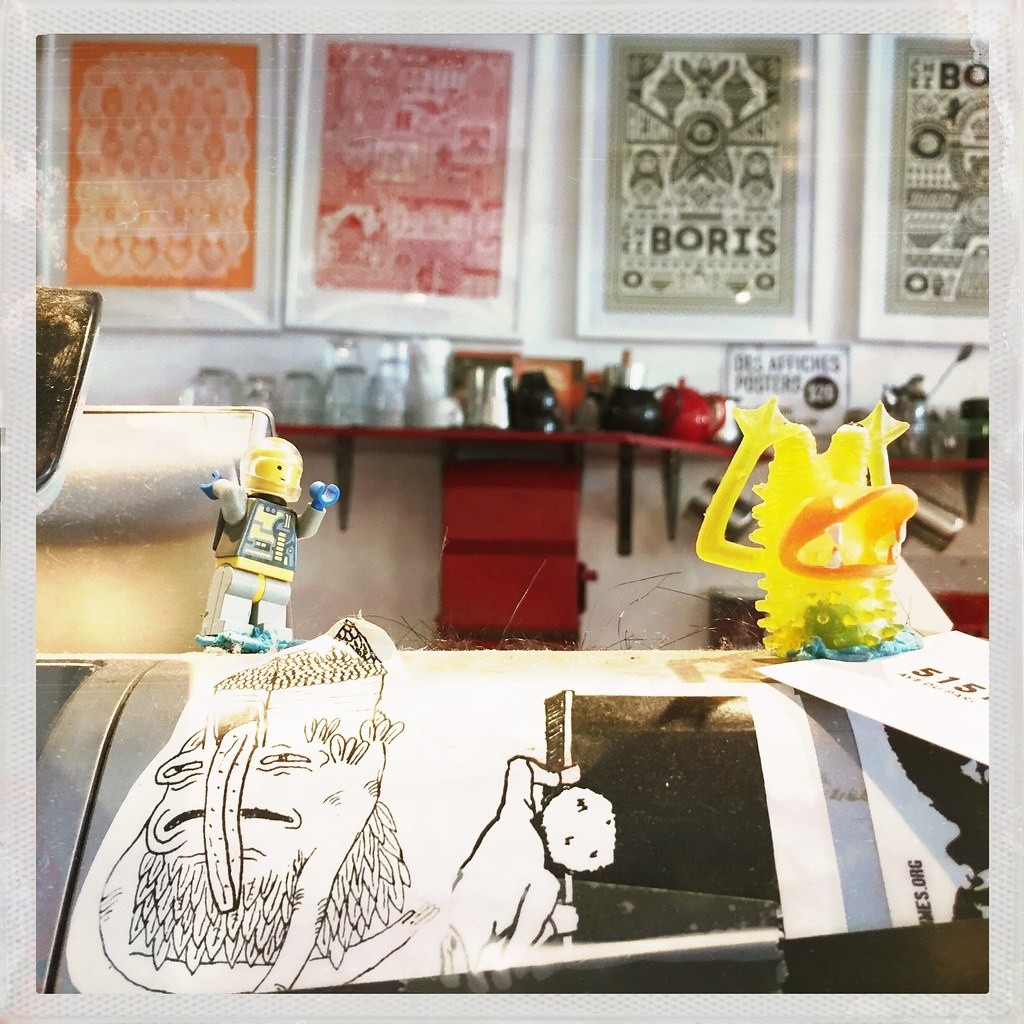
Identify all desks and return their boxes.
[37,650,990,994]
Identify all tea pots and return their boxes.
[587,383,681,435]
[658,375,727,443]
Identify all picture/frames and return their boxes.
[574,33,822,344]
[285,34,538,340]
[856,31,990,346]
[36,34,290,332]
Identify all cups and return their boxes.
[190,358,411,425]
[905,488,963,552]
[465,364,513,429]
[683,477,753,544]
